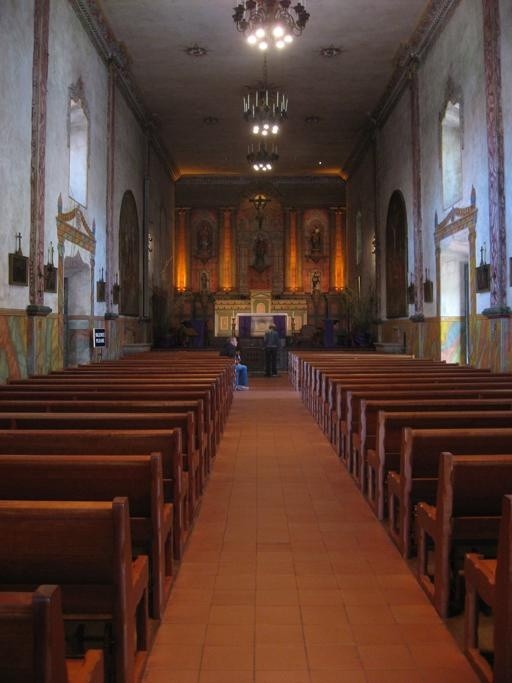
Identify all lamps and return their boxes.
[230,0,312,172]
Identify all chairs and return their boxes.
[416,451,512,619]
[0,343,241,532]
[288,350,512,560]
[1,494,153,682]
[2,450,174,619]
[461,493,512,683]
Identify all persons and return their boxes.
[219,337,249,390]
[264,324,281,376]
